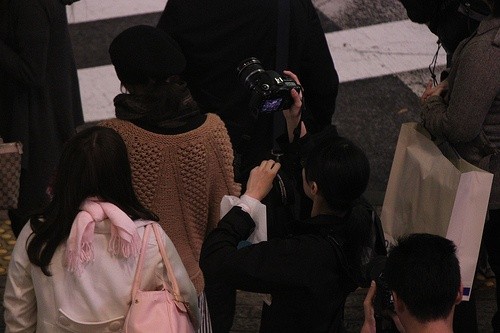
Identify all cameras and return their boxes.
[235,57,302,118]
[372,256,401,333]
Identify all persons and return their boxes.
[3,126,197,333]
[158,0,339,333]
[398,0,481,87]
[0,0,85,239]
[360,233,463,333]
[422,0,500,333]
[199,71,388,333]
[73,25,241,296]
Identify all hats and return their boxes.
[109,24,187,75]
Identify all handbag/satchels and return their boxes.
[379,122,494,303]
[123,223,196,332]
